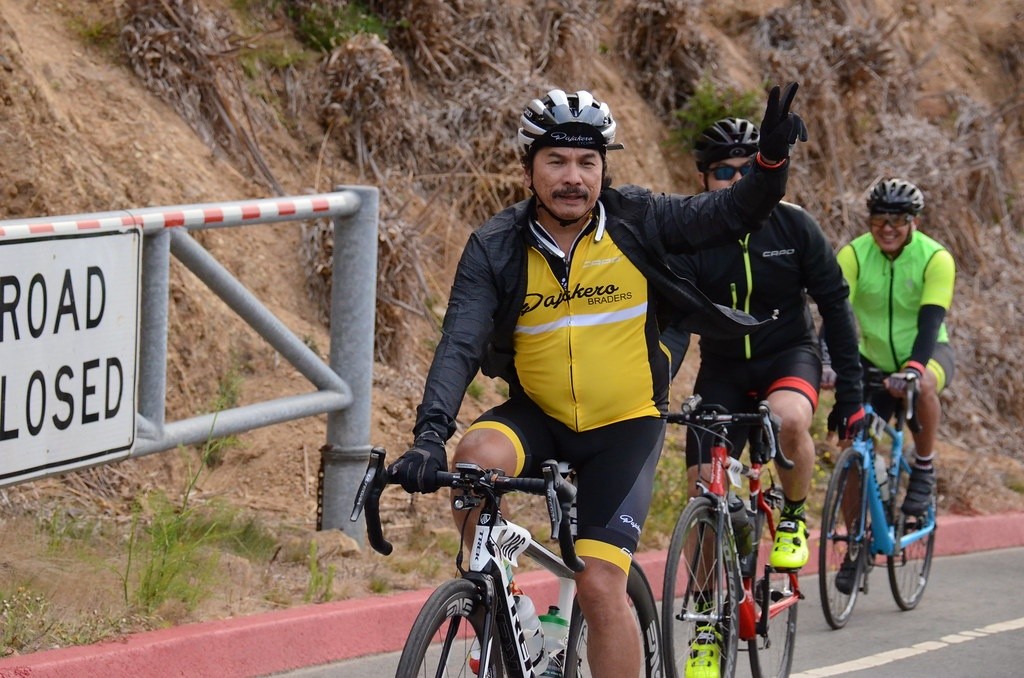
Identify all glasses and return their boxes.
[864,213,913,228]
[707,164,752,180]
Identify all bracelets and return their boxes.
[903,359,925,376]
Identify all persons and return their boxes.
[656,117,871,678]
[387,82,808,678]
[819,177,954,596]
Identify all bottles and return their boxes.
[512,594,549,676]
[873,454,890,501]
[739,512,757,572]
[885,466,898,508]
[534,606,569,678]
[728,491,752,557]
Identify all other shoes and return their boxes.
[468,587,526,674]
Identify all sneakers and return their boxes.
[769,504,810,571]
[834,551,858,594]
[900,473,934,515]
[684,625,724,678]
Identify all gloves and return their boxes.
[758,81,807,162]
[389,430,449,493]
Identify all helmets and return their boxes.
[517,90,617,167]
[867,179,925,216]
[694,117,761,172]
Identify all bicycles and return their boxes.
[661,395,806,677]
[347,445,667,678]
[817,367,938,630]
